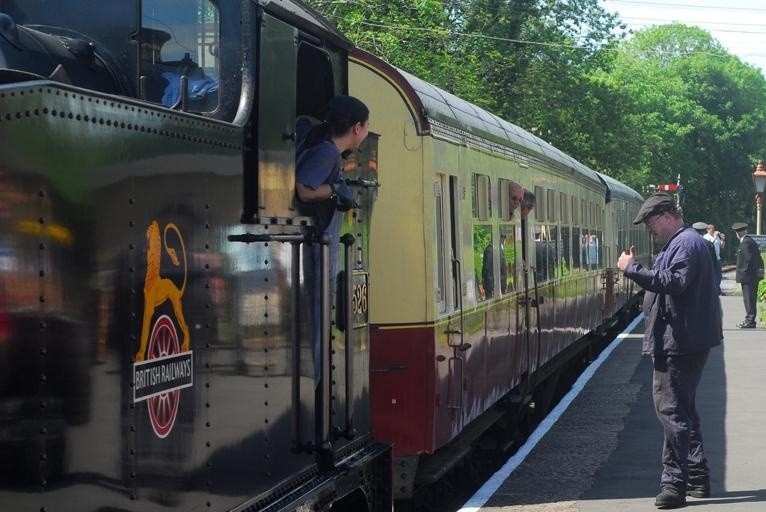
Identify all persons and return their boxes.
[731,222,764,328]
[616,191,723,506]
[691,222,724,340]
[521,188,535,220]
[703,223,725,298]
[293,96,370,391]
[482,233,510,298]
[579,233,600,269]
[507,180,523,212]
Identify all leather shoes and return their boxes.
[736,322,755,328]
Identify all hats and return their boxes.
[692,222,708,232]
[633,192,675,224]
[732,223,748,231]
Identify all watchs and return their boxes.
[327,182,338,203]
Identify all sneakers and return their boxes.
[687,485,706,497]
[655,489,687,507]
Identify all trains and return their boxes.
[1,0,658,510]
[750,157,766,234]
